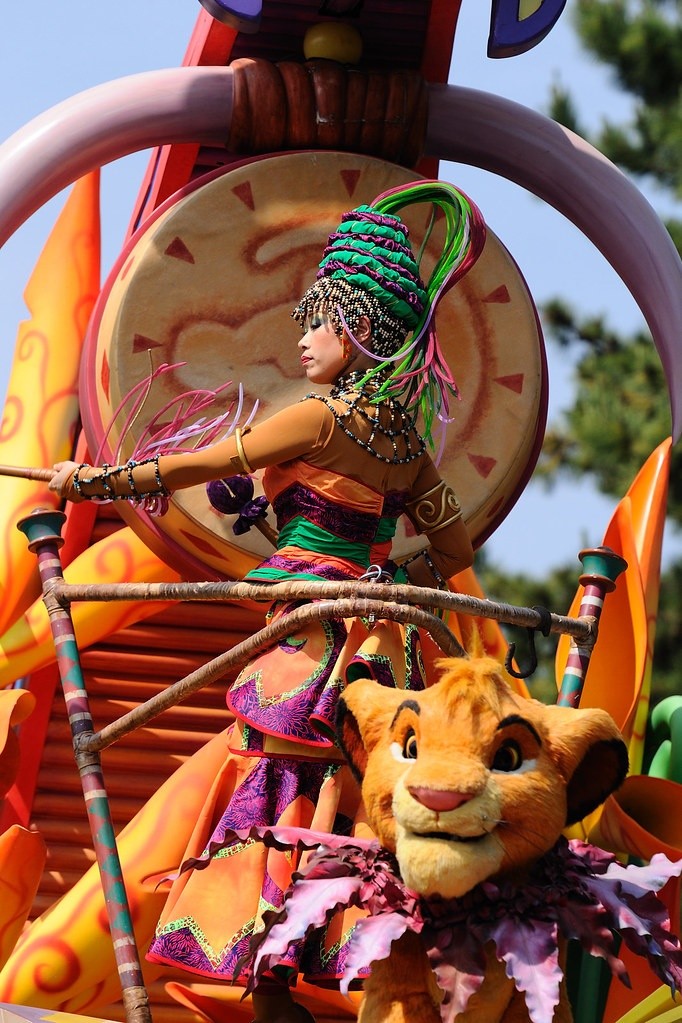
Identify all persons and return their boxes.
[50,177,682,1023]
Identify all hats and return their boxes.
[316,179,487,455]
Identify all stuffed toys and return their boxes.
[339,658,628,1023]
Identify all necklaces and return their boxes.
[302,368,425,464]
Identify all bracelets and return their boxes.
[74,452,168,501]
